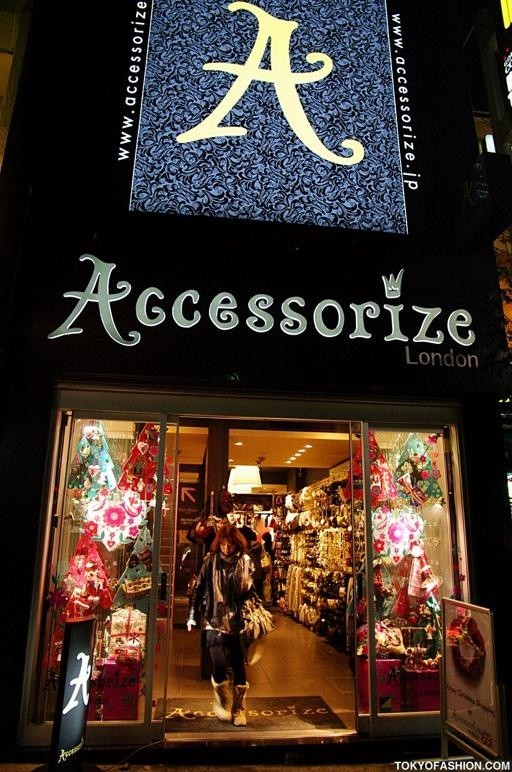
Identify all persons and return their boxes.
[186,525,261,726]
[240,525,270,606]
[262,532,275,561]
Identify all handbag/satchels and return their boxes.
[238,592,276,641]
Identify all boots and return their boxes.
[210,674,233,722]
[232,681,249,727]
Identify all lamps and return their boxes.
[232,465,262,486]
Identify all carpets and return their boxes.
[155,694,347,733]
